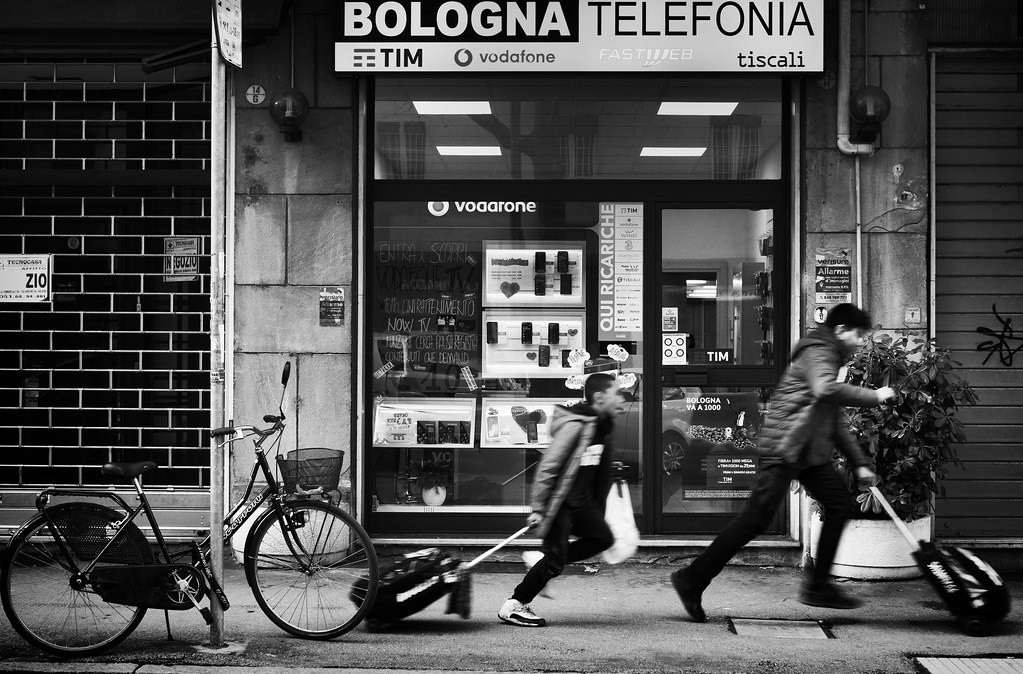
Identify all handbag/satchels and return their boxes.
[603,479,640,565]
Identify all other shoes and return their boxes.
[671,563,712,624]
[797,556,864,609]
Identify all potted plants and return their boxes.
[809,325,982,582]
[413,457,456,506]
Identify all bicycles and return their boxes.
[0,360,381,657]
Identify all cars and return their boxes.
[582,369,704,478]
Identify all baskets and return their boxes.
[276,447,344,495]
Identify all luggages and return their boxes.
[868,485,1013,636]
[349,519,540,632]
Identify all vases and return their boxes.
[231,504,353,567]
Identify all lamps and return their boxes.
[847,85,891,144]
[268,88,309,144]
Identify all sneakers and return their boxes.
[523,550,545,573]
[498,598,547,628]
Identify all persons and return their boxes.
[498,373,624,627]
[668,302,900,620]
[437,315,455,333]
[734,408,749,441]
